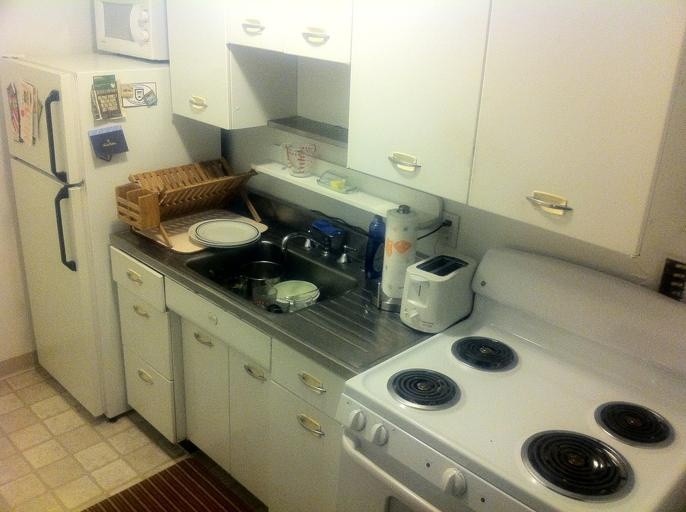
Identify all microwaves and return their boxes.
[94,0,169,60]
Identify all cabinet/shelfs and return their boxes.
[166,0,297,131]
[271,338,348,512]
[346,0,492,206]
[164,276,272,506]
[109,244,186,445]
[467,0,686,259]
[227,0,351,65]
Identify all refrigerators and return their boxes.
[0,52,221,423]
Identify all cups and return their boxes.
[285,143,316,178]
[252,285,278,309]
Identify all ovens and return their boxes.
[335,393,532,512]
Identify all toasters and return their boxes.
[400,254,477,334]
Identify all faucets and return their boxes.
[281,231,333,257]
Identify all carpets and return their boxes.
[69,454,265,512]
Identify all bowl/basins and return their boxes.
[248,261,282,284]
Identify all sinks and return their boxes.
[184,241,359,316]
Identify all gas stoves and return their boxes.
[350,322,685,512]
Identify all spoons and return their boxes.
[284,288,319,300]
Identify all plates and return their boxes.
[188,218,261,249]
[268,280,320,313]
